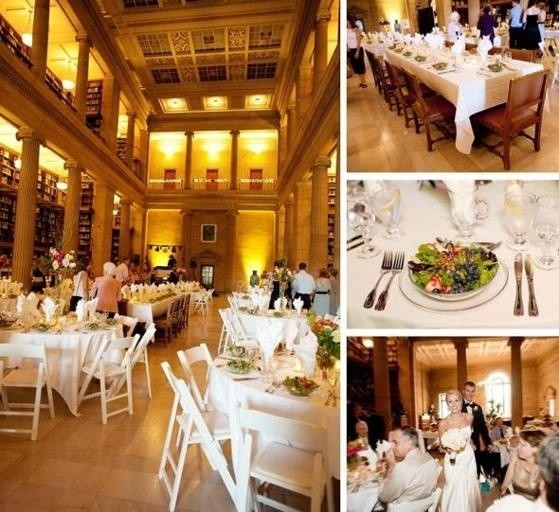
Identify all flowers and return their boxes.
[272,266,294,283]
[311,319,338,345]
[376,18,391,25]
[173,263,187,276]
[45,246,78,276]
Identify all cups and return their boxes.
[503,192,536,250]
[444,180,490,235]
[346,189,376,257]
[505,52,513,69]
[370,185,403,238]
[537,195,558,270]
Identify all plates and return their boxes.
[479,68,515,77]
[398,261,509,313]
[222,369,262,379]
[428,68,457,74]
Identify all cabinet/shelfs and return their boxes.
[86,80,102,118]
[79,213,90,254]
[0,14,31,68]
[111,228,134,263]
[35,205,56,251]
[328,214,334,260]
[117,138,127,159]
[328,176,336,206]
[0,191,17,247]
[81,176,92,207]
[0,144,21,187]
[46,67,77,114]
[37,167,58,201]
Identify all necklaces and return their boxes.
[448,414,468,423]
[523,464,531,473]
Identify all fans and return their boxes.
[49,44,79,68]
[6,0,55,25]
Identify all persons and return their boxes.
[438,390,482,512]
[434,11,438,26]
[378,426,443,512]
[250,271,260,287]
[169,269,179,284]
[284,282,292,310]
[522,0,542,50]
[508,0,523,49]
[489,417,511,441]
[477,6,498,46]
[116,256,131,284]
[501,430,548,504]
[314,268,331,315]
[347,15,367,88]
[348,420,377,456]
[31,258,45,294]
[460,382,495,481]
[70,257,91,311]
[269,260,282,309]
[152,274,158,283]
[394,20,402,34]
[128,256,140,287]
[486,436,559,512]
[262,270,270,279]
[330,269,339,315]
[168,255,177,268]
[539,2,546,43]
[89,262,124,318]
[140,263,153,285]
[291,262,317,311]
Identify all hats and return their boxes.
[253,270,257,272]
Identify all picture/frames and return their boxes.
[201,223,216,242]
[403,19,410,29]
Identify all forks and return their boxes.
[265,379,277,392]
[364,251,393,309]
[270,382,283,393]
[351,483,361,493]
[374,251,403,310]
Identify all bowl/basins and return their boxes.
[415,56,428,62]
[284,376,318,396]
[431,60,454,71]
[408,244,499,301]
[226,358,257,375]
[34,323,48,332]
[486,63,504,71]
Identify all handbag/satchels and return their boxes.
[480,471,491,493]
[70,271,84,311]
[372,501,387,512]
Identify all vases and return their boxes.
[179,275,182,281]
[383,26,386,33]
[55,272,73,314]
[279,283,285,310]
[317,347,331,371]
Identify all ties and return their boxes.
[500,426,504,439]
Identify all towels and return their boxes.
[274,297,287,311]
[414,38,421,52]
[293,297,303,312]
[428,37,440,54]
[478,35,492,59]
[494,37,501,48]
[27,292,39,313]
[75,298,88,320]
[286,321,299,350]
[158,282,175,291]
[131,283,157,296]
[258,335,282,361]
[17,293,25,312]
[40,297,55,322]
[121,285,129,299]
[86,299,98,319]
[444,180,475,224]
[295,333,319,362]
[177,280,199,289]
[363,180,384,197]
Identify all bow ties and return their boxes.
[465,403,473,408]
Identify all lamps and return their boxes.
[54,175,68,191]
[13,150,22,170]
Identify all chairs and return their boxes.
[176,344,213,447]
[184,294,190,328]
[193,294,207,317]
[505,49,534,63]
[162,298,185,338]
[114,313,138,338]
[232,291,238,295]
[204,289,215,307]
[387,488,442,512]
[109,323,157,399]
[445,41,454,47]
[0,343,54,441]
[227,297,238,309]
[151,300,175,348]
[544,38,558,49]
[401,67,456,151]
[365,49,379,90]
[384,61,437,128]
[466,44,477,50]
[158,362,238,512]
[471,70,549,170]
[76,334,140,425]
[538,40,559,89]
[117,301,145,351]
[218,308,239,353]
[374,59,406,111]
[369,52,391,99]
[229,402,333,512]
[488,47,502,55]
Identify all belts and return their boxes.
[296,293,309,296]
[316,292,328,294]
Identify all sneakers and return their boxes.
[360,82,367,88]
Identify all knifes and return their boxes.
[526,253,538,315]
[513,254,525,314]
